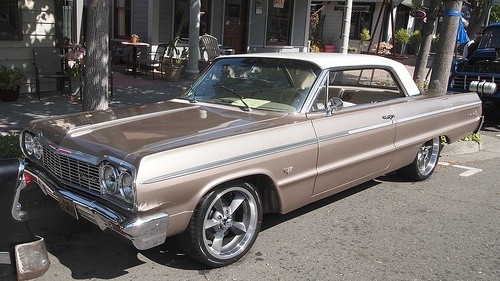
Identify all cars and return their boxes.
[20,52,483,267]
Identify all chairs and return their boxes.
[31,46,71,100]
[140,42,169,80]
[200,35,230,63]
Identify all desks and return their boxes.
[111,39,150,80]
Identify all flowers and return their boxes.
[64,42,86,71]
[371,41,393,55]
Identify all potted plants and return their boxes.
[0,64,26,103]
[162,35,198,82]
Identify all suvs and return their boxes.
[449,22,500,124]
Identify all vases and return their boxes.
[68,75,83,97]
